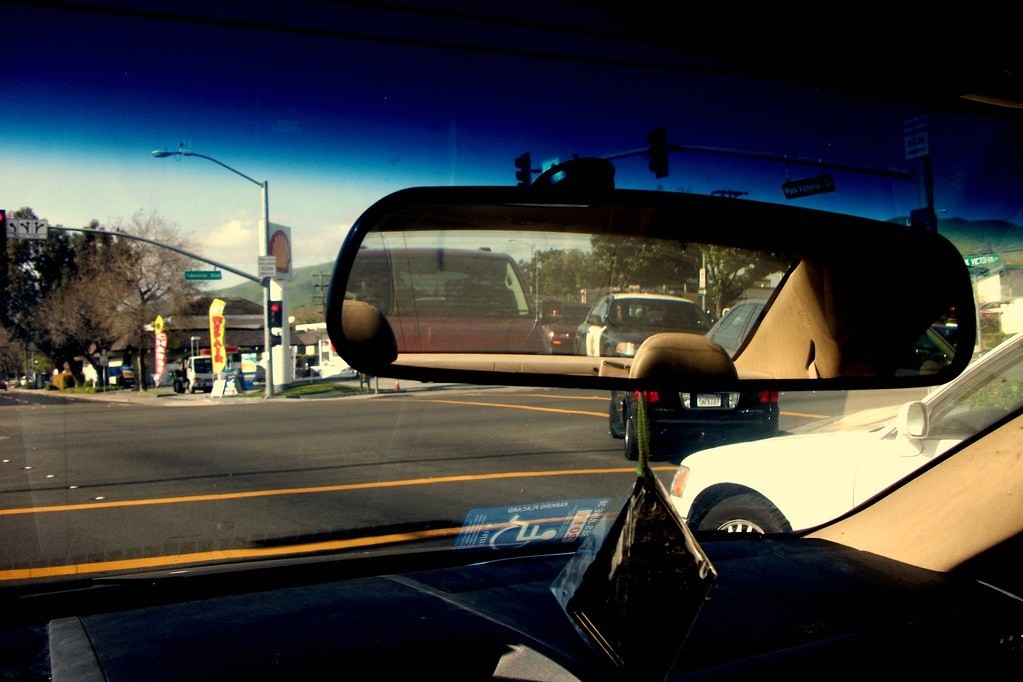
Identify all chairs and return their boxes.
[442,277,472,302]
[339,294,409,368]
[627,333,738,378]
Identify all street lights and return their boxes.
[151,149,274,400]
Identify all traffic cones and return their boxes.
[393,378,401,390]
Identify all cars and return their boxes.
[573,292,713,360]
[537,302,596,358]
[342,246,553,361]
[699,297,771,360]
[608,388,783,462]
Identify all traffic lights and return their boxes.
[0,209,7,256]
[647,124,669,181]
[268,300,282,328]
[513,151,531,188]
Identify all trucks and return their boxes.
[169,354,214,395]
